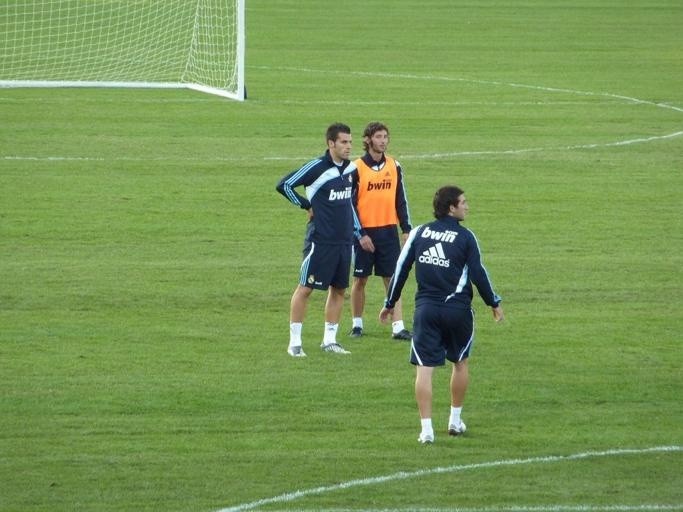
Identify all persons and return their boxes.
[274,122,360,357]
[335,122,415,343]
[376,185,506,444]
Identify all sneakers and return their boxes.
[350,326,362,337]
[418,432,434,443]
[321,342,351,354]
[448,420,466,435]
[392,329,411,340]
[287,345,307,356]
